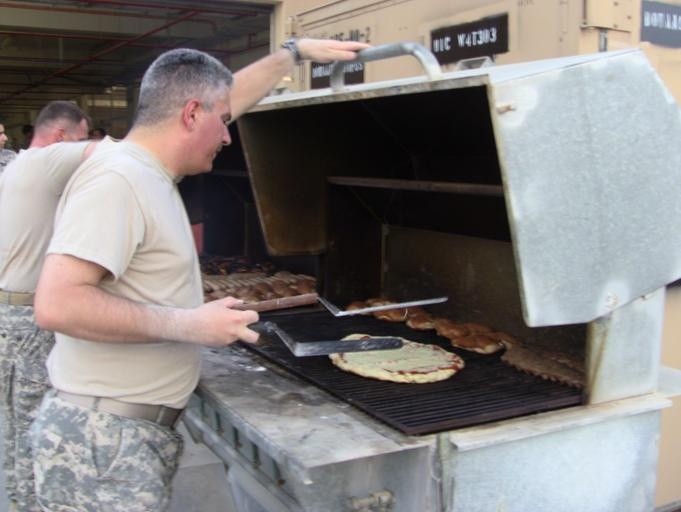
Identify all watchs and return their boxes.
[282,39,305,65]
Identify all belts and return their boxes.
[57,391,187,429]
[1,291,34,306]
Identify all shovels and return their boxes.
[229,292,449,318]
[247,321,404,358]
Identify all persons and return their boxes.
[29,37,375,511]
[0,100,99,512]
